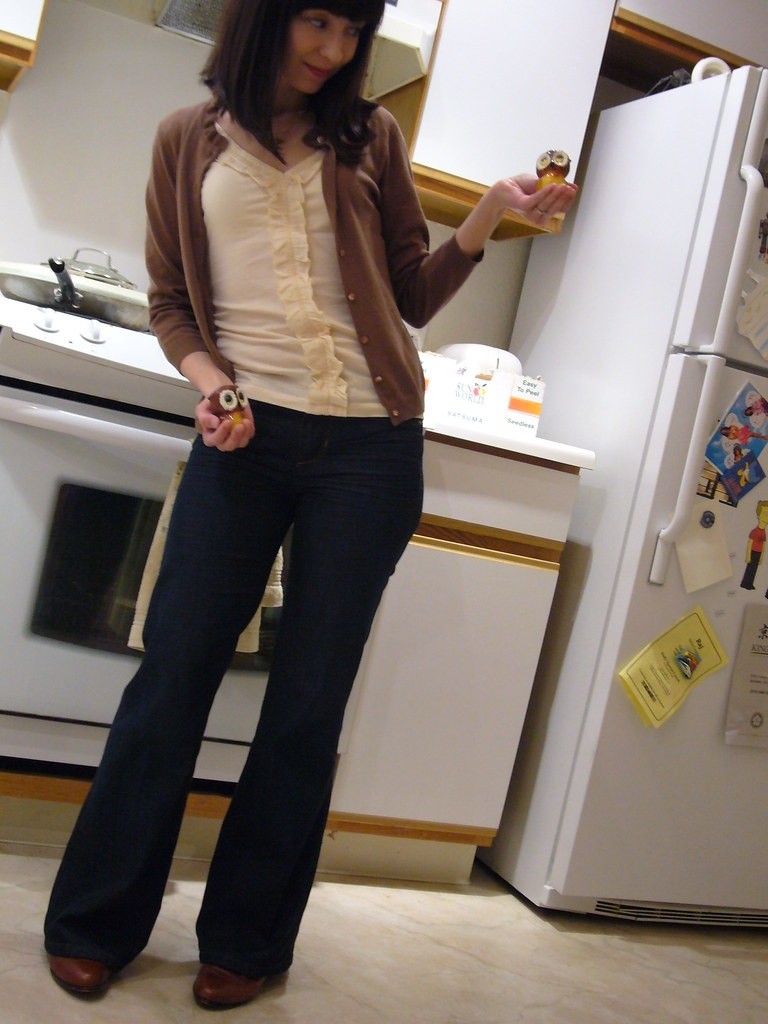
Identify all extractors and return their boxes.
[76,0,444,103]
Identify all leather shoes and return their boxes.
[46,951,114,992]
[191,962,268,1009]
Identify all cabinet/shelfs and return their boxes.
[329,436,579,829]
[601,0,768,93]
[376,0,618,241]
[0,0,50,93]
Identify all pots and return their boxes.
[0,247,157,333]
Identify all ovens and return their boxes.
[0,384,353,792]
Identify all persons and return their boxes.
[41,0,577,1009]
[720,397,768,464]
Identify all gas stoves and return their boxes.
[0,295,213,419]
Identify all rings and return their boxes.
[535,207,544,214]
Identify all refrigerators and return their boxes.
[475,56,768,934]
